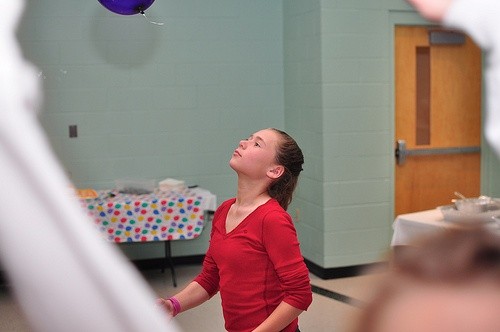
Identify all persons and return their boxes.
[357,227,500,332]
[158,128,313,332]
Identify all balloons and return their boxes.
[98,0,155,16]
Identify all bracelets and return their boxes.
[168,298,180,317]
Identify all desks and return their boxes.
[70,181,216,289]
[386,204,500,256]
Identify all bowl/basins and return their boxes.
[452,199,487,211]
